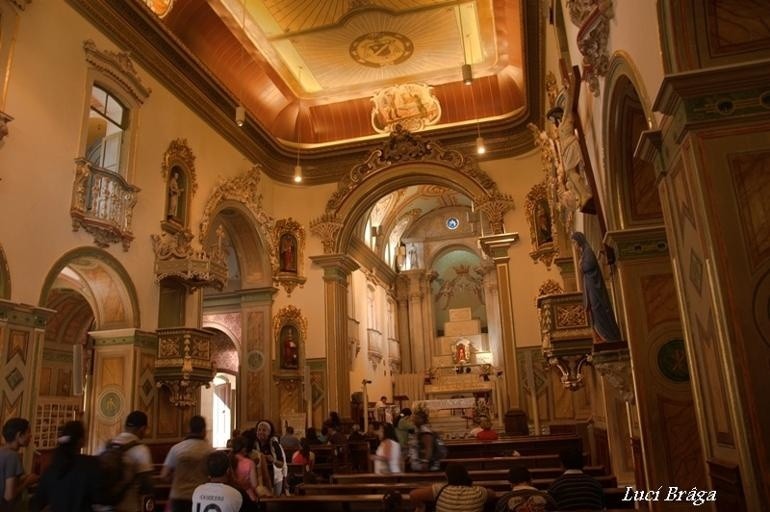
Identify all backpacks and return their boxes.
[90,437,147,506]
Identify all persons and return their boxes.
[534,203,554,242]
[280,233,295,272]
[169,170,186,219]
[571,231,619,342]
[93,408,157,509]
[31,419,127,511]
[1,417,43,510]
[457,345,466,361]
[162,392,440,512]
[281,326,301,369]
[409,461,496,512]
[539,72,595,217]
[469,397,492,429]
[547,445,609,512]
[493,463,558,512]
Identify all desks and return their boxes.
[417,397,476,417]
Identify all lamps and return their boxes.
[234,0,246,129]
[293,65,305,183]
[456,3,473,86]
[470,81,486,154]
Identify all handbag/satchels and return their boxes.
[418,431,447,463]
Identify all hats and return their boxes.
[186,415,205,434]
[125,410,148,427]
